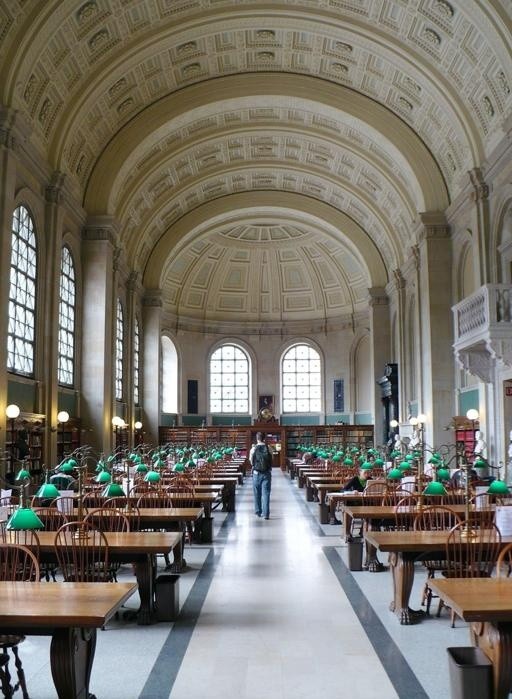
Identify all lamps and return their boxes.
[409,417,419,440]
[134,421,143,444]
[59,411,70,463]
[417,414,427,473]
[389,419,398,440]
[6,404,20,483]
[118,419,125,449]
[467,409,479,451]
[111,416,121,460]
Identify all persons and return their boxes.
[508,430,512,458]
[386,431,397,464]
[408,427,421,467]
[249,432,273,519]
[340,467,384,526]
[475,431,488,462]
[393,434,404,469]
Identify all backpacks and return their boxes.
[251,444,272,474]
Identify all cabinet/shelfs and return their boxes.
[7,412,47,496]
[58,417,81,467]
[159,425,373,468]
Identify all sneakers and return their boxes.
[255,511,262,517]
[264,518,269,519]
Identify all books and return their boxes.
[32,436,41,446]
[6,460,14,473]
[30,461,40,470]
[30,448,42,459]
